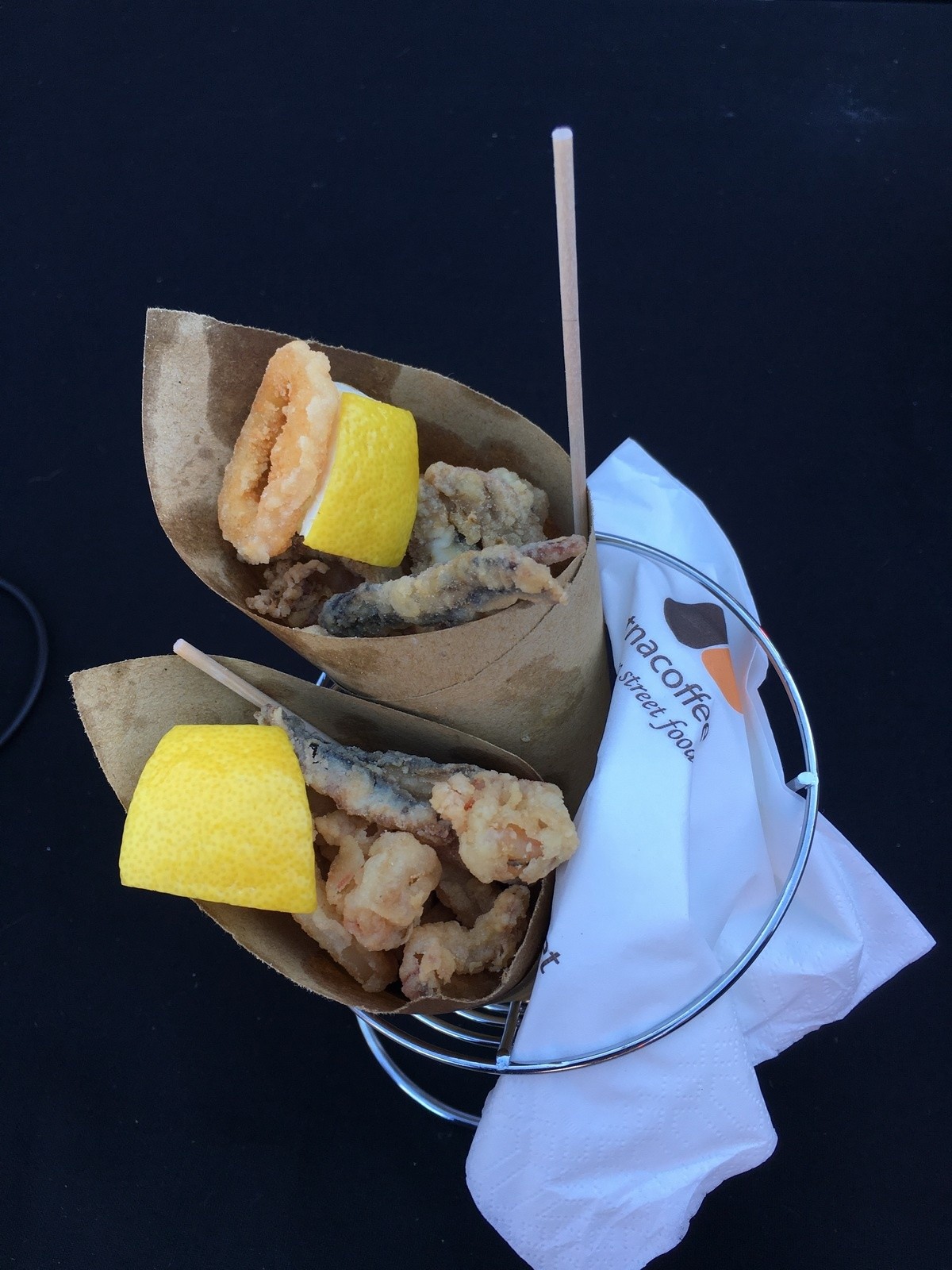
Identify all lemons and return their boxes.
[297,382,418,569]
[118,724,319,916]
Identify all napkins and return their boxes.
[466,435,938,1270]
